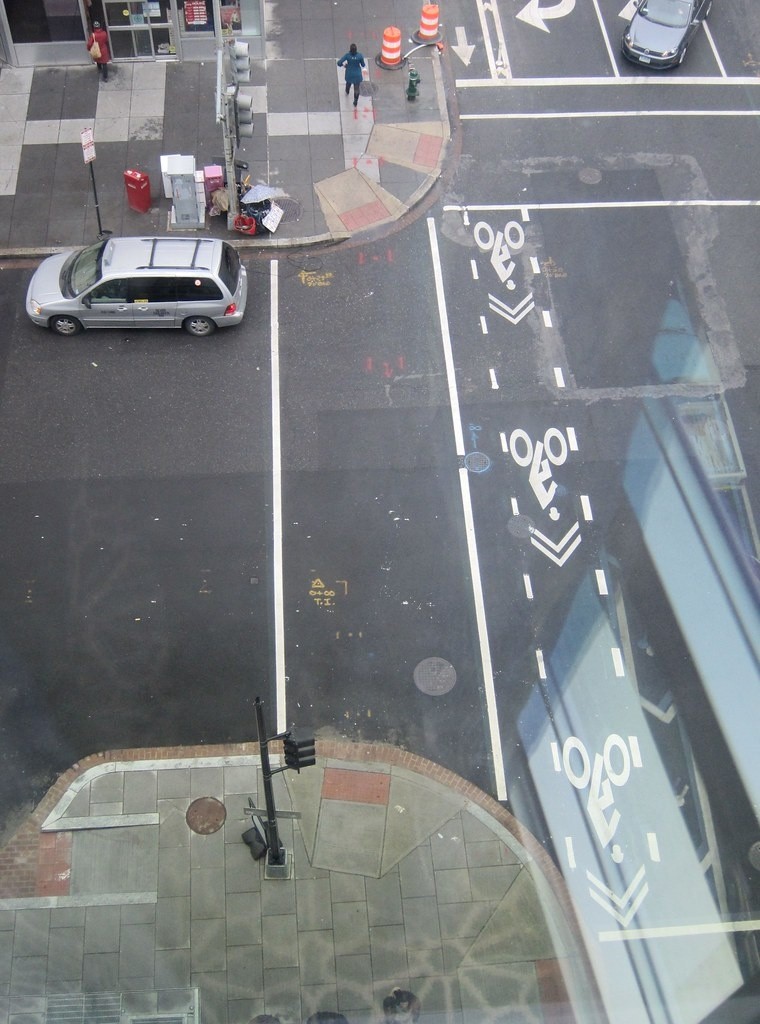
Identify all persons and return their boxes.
[338,44,365,107]
[87,21,110,82]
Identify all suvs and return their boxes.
[25,236,248,337]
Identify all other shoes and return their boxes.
[98,66,102,69]
[103,77,108,82]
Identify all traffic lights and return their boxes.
[229,39,252,85]
[283,736,317,774]
[229,86,254,147]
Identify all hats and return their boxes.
[93,21,100,28]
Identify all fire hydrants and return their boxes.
[406,70,421,101]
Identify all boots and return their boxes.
[345,85,350,94]
[353,93,359,107]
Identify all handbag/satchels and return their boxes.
[89,33,102,59]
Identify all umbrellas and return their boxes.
[241,185,275,205]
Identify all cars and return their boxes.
[620,0,712,72]
